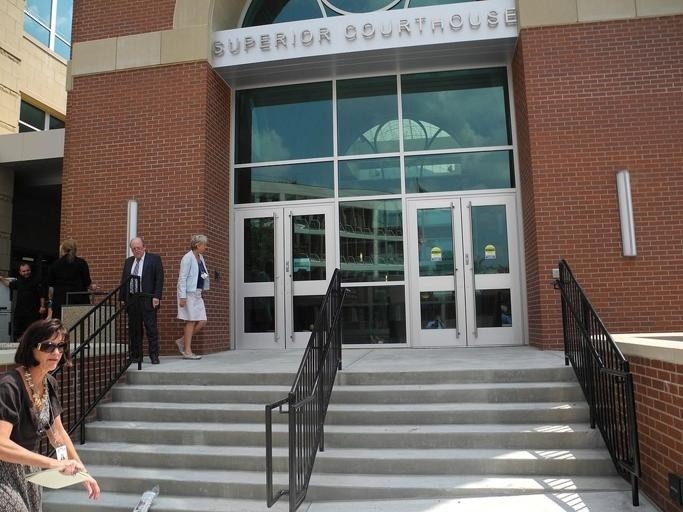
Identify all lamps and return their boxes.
[124,200,139,259]
[614,169,638,258]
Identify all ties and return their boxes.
[130,259,140,294]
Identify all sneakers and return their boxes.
[182,353,202,360]
[174,338,184,355]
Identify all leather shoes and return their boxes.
[132,356,143,364]
[150,356,161,365]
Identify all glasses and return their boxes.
[33,342,68,352]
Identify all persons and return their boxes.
[43,238,97,322]
[170,234,210,359]
[0,319,99,512]
[0,259,46,343]
[117,237,163,365]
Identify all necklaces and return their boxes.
[21,364,48,413]
